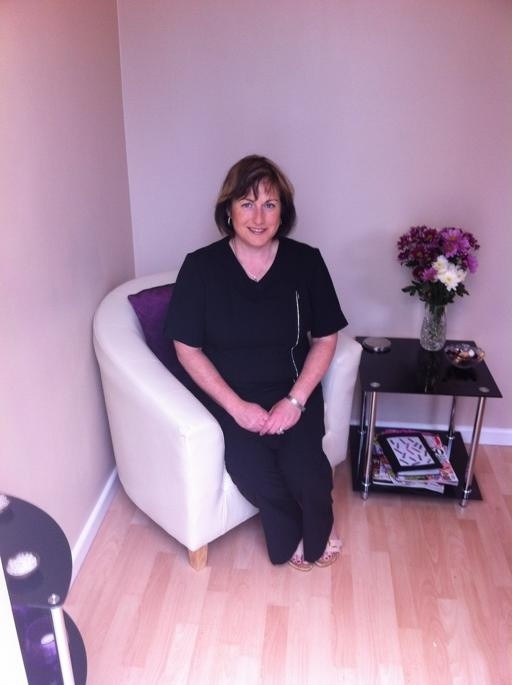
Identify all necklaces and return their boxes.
[243,264,258,281]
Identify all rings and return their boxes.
[279,428,284,432]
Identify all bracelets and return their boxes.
[286,395,305,412]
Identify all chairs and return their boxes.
[90,268,363,570]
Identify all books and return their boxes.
[378,433,443,476]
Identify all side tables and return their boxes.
[349,334,503,506]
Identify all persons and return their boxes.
[164,153,349,572]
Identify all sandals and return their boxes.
[288,540,341,571]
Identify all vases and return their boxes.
[419,303,448,352]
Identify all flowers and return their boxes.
[395,225,482,338]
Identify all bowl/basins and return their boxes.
[443,343,485,369]
